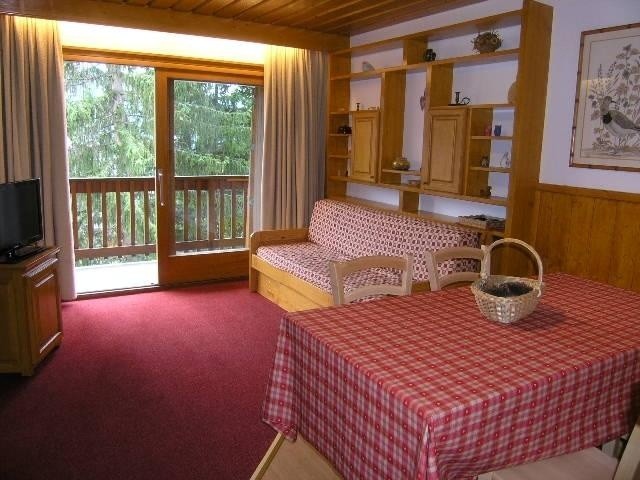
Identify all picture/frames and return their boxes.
[569,22,640,172]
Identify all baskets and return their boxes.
[471,237,546,324]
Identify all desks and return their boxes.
[263,272,640,480]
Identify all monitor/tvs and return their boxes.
[1,176,44,264]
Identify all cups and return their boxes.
[494,125,501,136]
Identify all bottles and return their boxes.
[481,155,490,168]
[501,151,511,168]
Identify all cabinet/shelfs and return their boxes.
[0,244,64,376]
[323,0,554,276]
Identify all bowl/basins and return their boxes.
[408,180,421,187]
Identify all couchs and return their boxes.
[248,199,493,313]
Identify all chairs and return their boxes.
[329,252,413,305]
[478,422,639,478]
[423,244,492,291]
